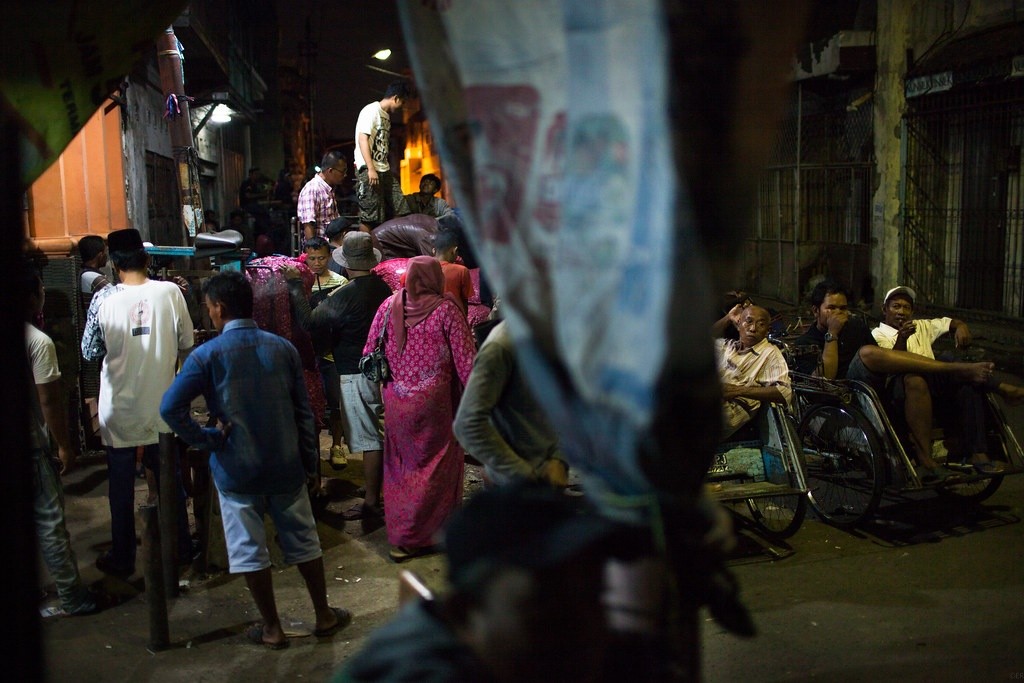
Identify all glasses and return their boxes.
[327,167,349,175]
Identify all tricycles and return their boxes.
[705,323,1024,541]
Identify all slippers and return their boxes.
[316,607,351,635]
[943,456,974,469]
[337,503,384,521]
[348,487,384,501]
[245,624,289,650]
[388,541,438,560]
[976,459,1004,475]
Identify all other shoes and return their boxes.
[95,551,129,575]
[907,467,960,485]
[178,563,192,591]
[40,601,96,618]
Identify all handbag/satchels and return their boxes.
[359,353,389,382]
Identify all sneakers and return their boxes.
[330,445,348,467]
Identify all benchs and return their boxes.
[702,393,804,506]
[777,332,983,448]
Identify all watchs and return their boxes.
[824,332,838,342]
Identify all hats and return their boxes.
[326,217,360,238]
[883,286,916,310]
[331,231,381,270]
[445,478,657,584]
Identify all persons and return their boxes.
[160,270,351,650]
[396,174,452,216]
[353,81,410,231]
[272,168,292,211]
[794,280,994,479]
[431,231,475,317]
[326,479,654,683]
[297,151,349,243]
[872,286,1024,474]
[81,229,194,575]
[372,213,459,261]
[281,230,393,520]
[361,254,477,562]
[19,246,105,614]
[325,216,349,281]
[453,318,571,488]
[712,303,792,442]
[205,210,245,248]
[77,235,110,293]
[306,236,350,417]
[239,168,270,225]
